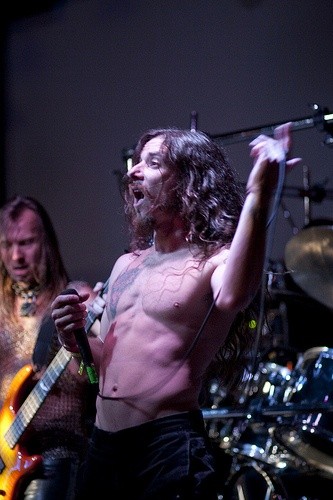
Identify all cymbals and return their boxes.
[285,226,333,309]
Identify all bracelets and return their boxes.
[58,332,81,358]
[245,187,275,196]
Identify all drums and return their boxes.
[226,363,314,473]
[274,347,333,473]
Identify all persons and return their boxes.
[51,122,302,500]
[0,195,108,500]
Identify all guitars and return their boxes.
[0,278,109,500]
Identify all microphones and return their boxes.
[58,289,100,394]
